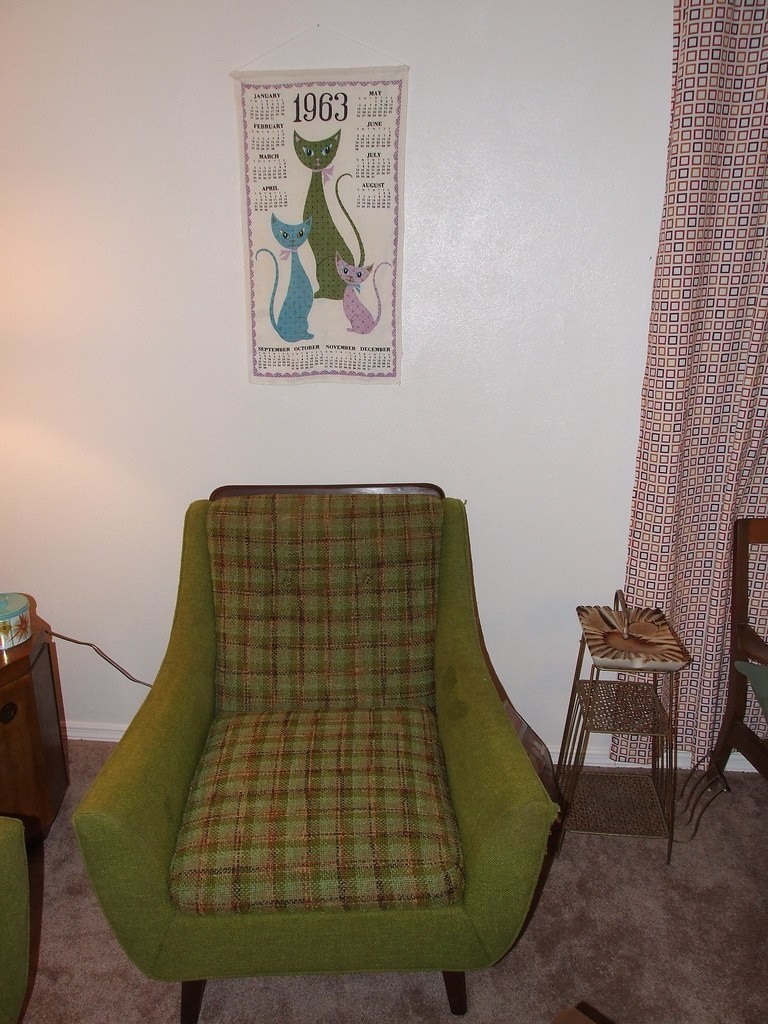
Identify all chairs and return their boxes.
[73,482,568,1024]
[708,518,768,786]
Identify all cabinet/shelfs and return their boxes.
[0,615,70,842]
[552,590,730,864]
